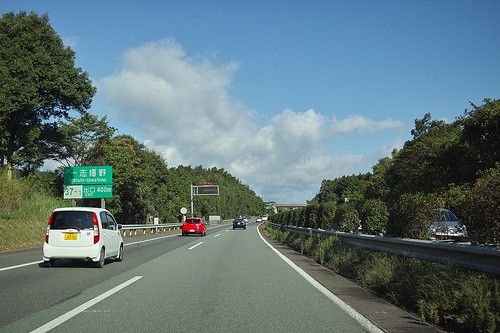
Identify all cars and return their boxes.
[427,207,467,241]
[255,215,268,223]
[232,218,247,230]
[181,217,208,237]
[41,205,125,268]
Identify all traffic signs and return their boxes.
[63,165,113,185]
